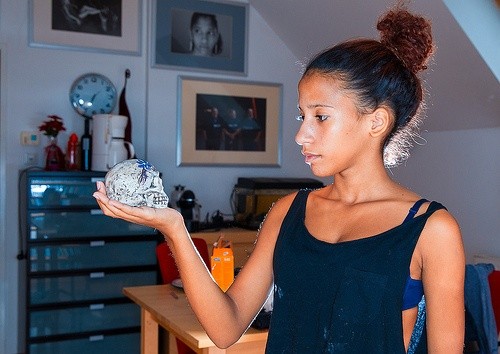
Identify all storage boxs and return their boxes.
[234,176,325,225]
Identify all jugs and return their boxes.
[107,138,134,169]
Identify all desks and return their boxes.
[122,284,270,354]
[189,228,259,271]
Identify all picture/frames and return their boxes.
[151,0,250,77]
[27,0,144,56]
[175,74,283,168]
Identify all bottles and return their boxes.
[67,133,81,169]
[170,184,186,213]
[211,248,235,293]
[80,117,92,172]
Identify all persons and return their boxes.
[202,107,262,151]
[190,12,224,56]
[63,0,120,35]
[92,0,465,354]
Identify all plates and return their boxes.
[171,279,182,288]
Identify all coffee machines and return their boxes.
[91,114,135,172]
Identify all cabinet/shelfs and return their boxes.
[17,169,164,354]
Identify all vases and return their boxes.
[43,136,67,171]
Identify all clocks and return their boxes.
[70,74,117,119]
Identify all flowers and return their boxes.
[38,114,66,146]
